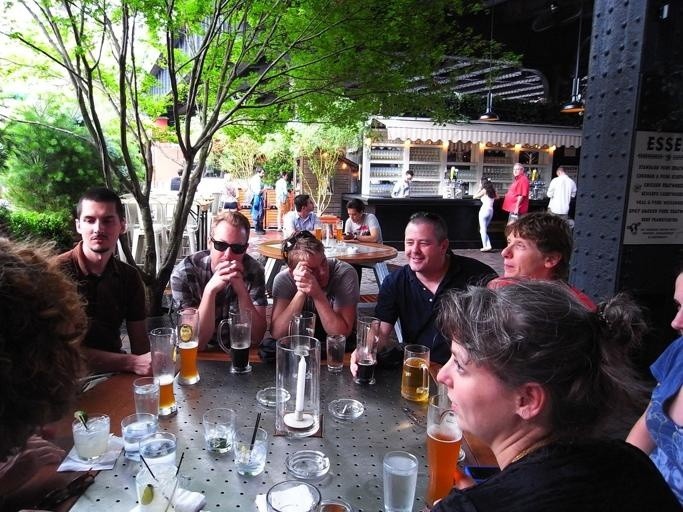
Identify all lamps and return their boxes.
[478,0,498,123]
[559,1,586,113]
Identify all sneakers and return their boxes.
[480,248,488,252]
[487,247,492,251]
[257,231,265,234]
[262,229,265,231]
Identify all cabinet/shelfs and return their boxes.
[447,141,478,194]
[482,142,554,198]
[361,137,443,197]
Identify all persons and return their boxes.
[275,172,291,231]
[481,212,594,317]
[282,195,321,240]
[50,187,175,377]
[250,167,268,234]
[171,170,182,191]
[428,275,683,512]
[349,213,500,379]
[169,211,268,352]
[270,232,359,352]
[344,199,382,243]
[390,169,414,198]
[626,271,683,507]
[474,177,500,252]
[1,230,95,512]
[220,173,239,213]
[548,166,576,216]
[501,164,530,226]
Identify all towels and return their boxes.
[255,484,318,512]
[129,487,207,512]
[57,436,125,473]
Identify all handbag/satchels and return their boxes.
[507,211,519,226]
[225,185,242,210]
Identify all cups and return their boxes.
[132,376,160,421]
[315,227,322,240]
[216,307,253,375]
[135,462,179,512]
[352,316,381,387]
[325,334,347,373]
[138,431,177,470]
[288,310,316,380]
[120,412,158,462]
[426,396,463,506]
[266,481,322,512]
[322,224,330,248]
[203,407,236,455]
[402,345,431,402]
[320,498,354,512]
[71,410,110,461]
[382,450,419,512]
[427,393,451,430]
[232,426,269,477]
[335,219,344,244]
[175,307,201,386]
[149,327,178,416]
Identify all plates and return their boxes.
[345,240,358,243]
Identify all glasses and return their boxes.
[513,168,522,170]
[210,235,249,254]
[35,471,95,511]
[283,230,313,265]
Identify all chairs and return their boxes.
[164,198,199,260]
[129,198,164,274]
